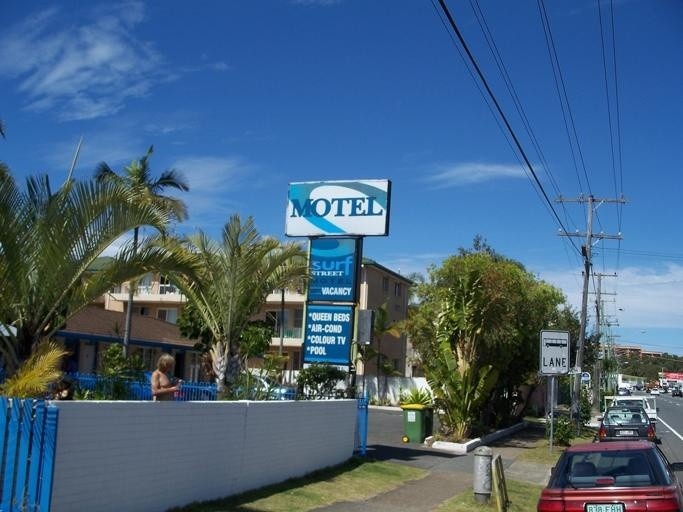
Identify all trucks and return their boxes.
[603,396,657,430]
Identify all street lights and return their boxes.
[616,361,629,383]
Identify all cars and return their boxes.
[645,381,682,397]
[582,372,590,385]
[616,386,632,396]
[192,374,294,402]
[537,440,682,512]
[597,406,658,442]
[128,370,191,403]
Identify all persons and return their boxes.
[55,389,73,401]
[149,354,181,400]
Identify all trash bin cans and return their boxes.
[425,404,433,438]
[400,403,428,444]
